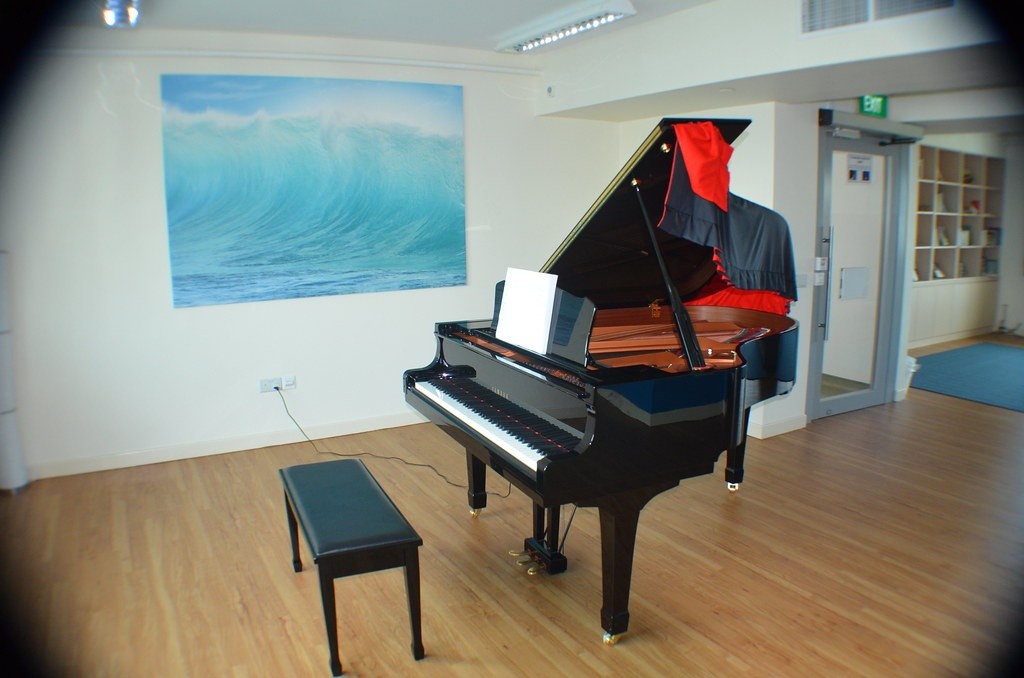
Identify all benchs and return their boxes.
[277,457,428,677]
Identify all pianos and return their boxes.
[402,115,800,645]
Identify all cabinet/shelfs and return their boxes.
[906,141,1007,285]
[906,281,998,351]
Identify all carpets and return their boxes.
[908,341,1023,412]
